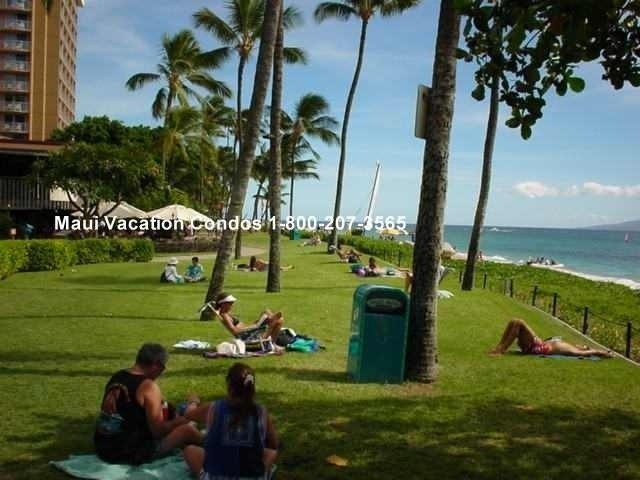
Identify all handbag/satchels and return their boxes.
[276,327,299,346]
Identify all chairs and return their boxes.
[199,299,278,355]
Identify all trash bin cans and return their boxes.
[289,228,300,241]
[352,229,363,236]
[347,284,407,384]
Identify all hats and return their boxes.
[166,257,179,265]
[217,294,237,305]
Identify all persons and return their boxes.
[93,341,208,466]
[336,243,362,261]
[249,255,296,273]
[363,257,380,276]
[182,256,208,282]
[179,361,282,480]
[485,317,617,359]
[302,231,322,246]
[215,290,287,352]
[163,257,185,284]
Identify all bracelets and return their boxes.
[189,401,199,405]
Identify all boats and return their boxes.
[527,260,564,268]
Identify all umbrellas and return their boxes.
[68,199,215,239]
